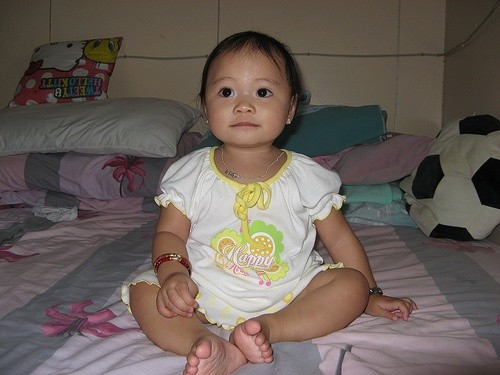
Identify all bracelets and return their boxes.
[369,287,383,295]
[153,253,191,277]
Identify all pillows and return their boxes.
[401,112,500,244]
[6,36,128,108]
[0,134,200,197]
[332,132,433,185]
[0,99,202,158]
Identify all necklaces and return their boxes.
[221,145,285,179]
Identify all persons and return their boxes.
[121,30,419,375]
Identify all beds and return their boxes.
[0,188,500,372]
